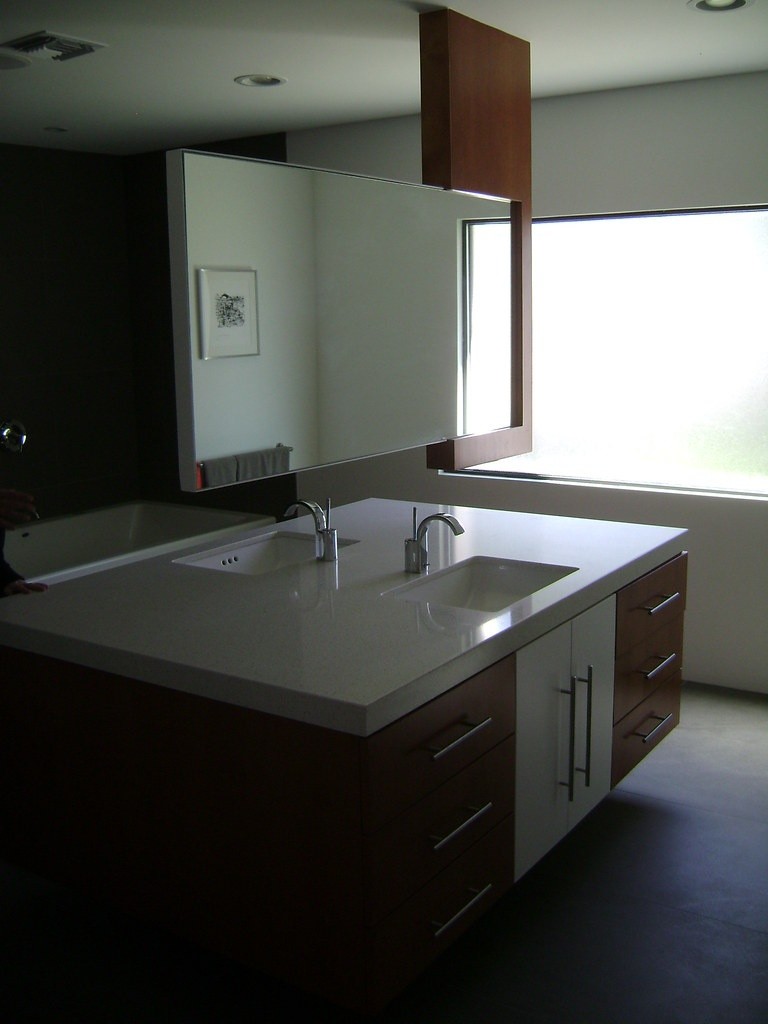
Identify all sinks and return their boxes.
[171,530,361,576]
[381,556,580,613]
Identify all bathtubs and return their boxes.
[0,496,276,582]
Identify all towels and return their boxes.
[195,442,290,490]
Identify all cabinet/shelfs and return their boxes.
[2,497,695,991]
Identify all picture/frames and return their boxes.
[196,269,263,364]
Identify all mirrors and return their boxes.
[164,149,528,493]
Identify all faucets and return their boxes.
[284,497,339,561]
[404,506,466,575]
